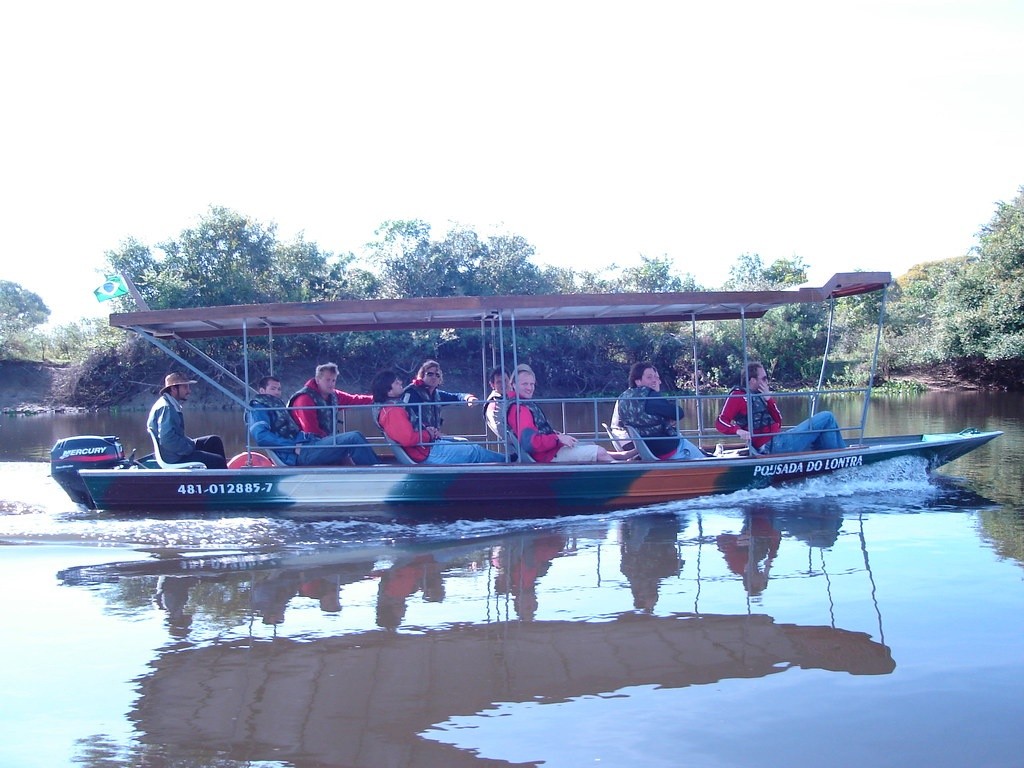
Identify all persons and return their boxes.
[145,373,228,469]
[156,511,781,640]
[243,359,846,465]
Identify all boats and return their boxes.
[40,268,1005,525]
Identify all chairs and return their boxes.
[603,423,640,461]
[743,436,761,455]
[382,429,418,466]
[625,424,659,462]
[505,430,536,464]
[148,427,206,468]
[264,448,289,467]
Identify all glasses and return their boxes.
[423,372,441,378]
[756,375,767,382]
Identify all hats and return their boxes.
[510,364,534,383]
[159,371,197,394]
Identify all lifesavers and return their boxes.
[227,450,272,469]
